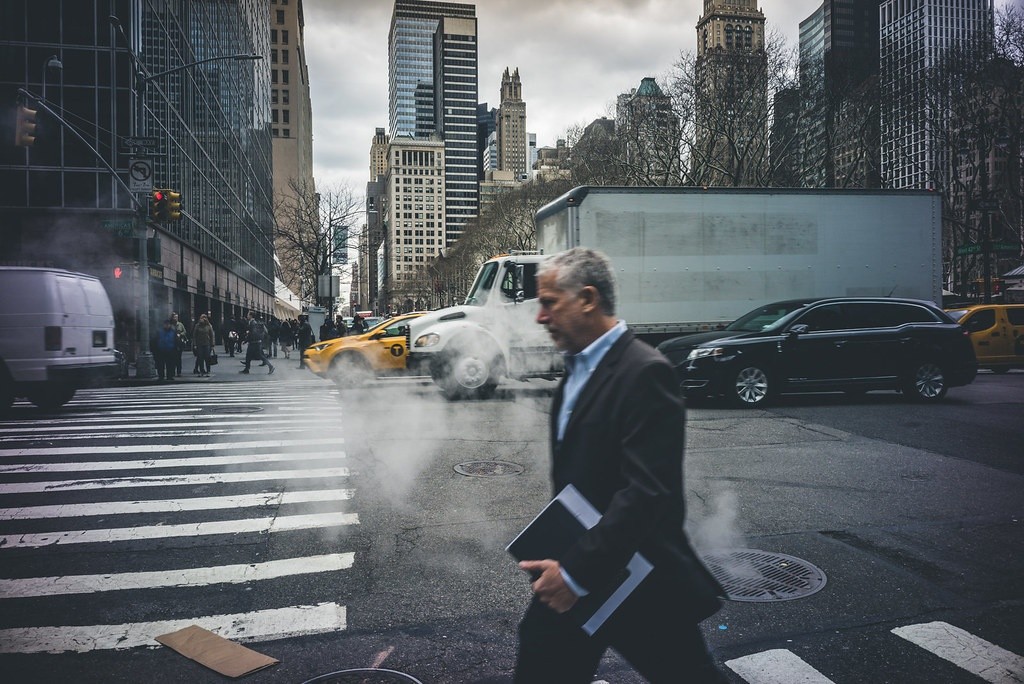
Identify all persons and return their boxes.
[513,246,732,684]
[149,309,389,384]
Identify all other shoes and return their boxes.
[193,369,216,377]
[295,366,305,369]
[178,373,183,377]
[240,360,246,365]
[230,354,235,357]
[157,377,175,381]
[239,370,249,374]
[259,362,267,366]
[268,366,276,375]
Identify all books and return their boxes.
[502,495,633,631]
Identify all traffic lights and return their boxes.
[13,103,39,148]
[149,188,183,225]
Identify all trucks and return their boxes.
[404,186,945,396]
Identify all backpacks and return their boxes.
[258,321,268,340]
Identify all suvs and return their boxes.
[342,317,355,330]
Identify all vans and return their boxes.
[943,302,1024,373]
[0,265,117,420]
[303,311,434,390]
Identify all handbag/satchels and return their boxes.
[229,331,239,342]
[310,327,316,344]
[210,349,218,365]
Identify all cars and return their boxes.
[361,317,386,333]
[655,297,979,411]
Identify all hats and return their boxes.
[351,314,365,323]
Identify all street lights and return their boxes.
[134,53,265,380]
